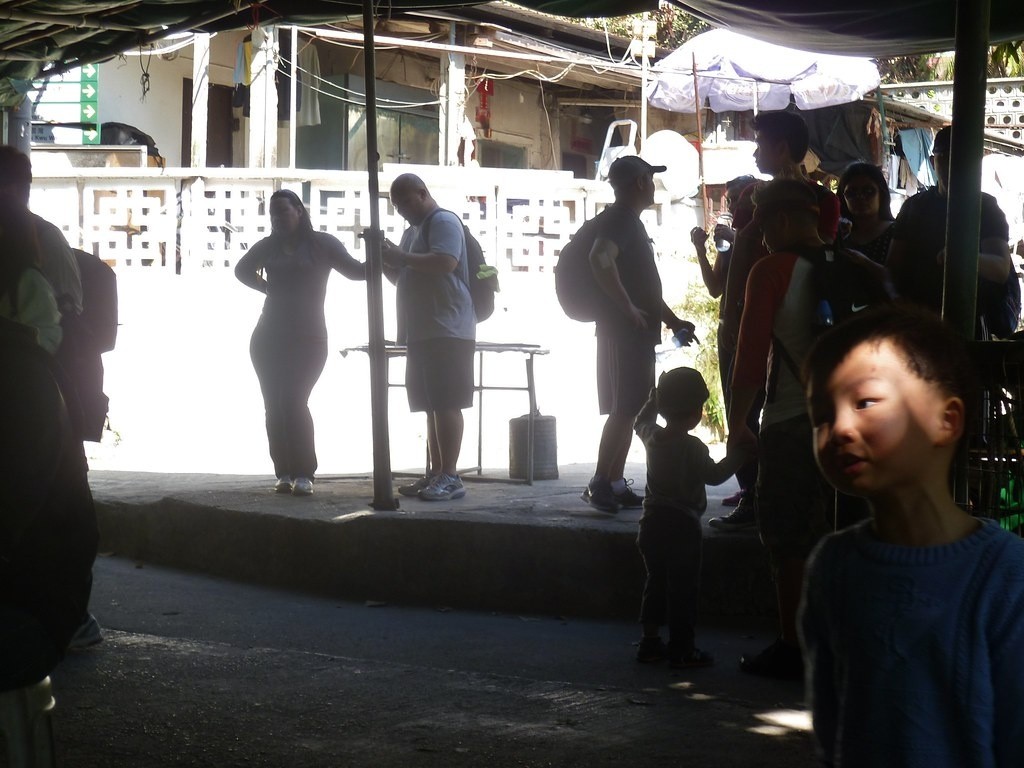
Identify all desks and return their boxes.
[348,343,551,488]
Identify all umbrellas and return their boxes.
[647,29,881,140]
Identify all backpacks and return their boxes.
[766,246,887,381]
[422,209,501,324]
[556,203,645,324]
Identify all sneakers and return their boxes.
[579,476,647,516]
[397,472,468,501]
[706,499,755,531]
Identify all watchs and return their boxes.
[399,252,407,267]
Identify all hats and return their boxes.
[607,156,667,192]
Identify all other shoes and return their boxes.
[738,636,797,673]
[274,474,294,495]
[633,636,676,666]
[670,642,717,670]
[291,475,316,497]
[68,614,104,648]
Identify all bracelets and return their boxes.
[667,317,679,328]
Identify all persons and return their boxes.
[556,157,699,513]
[887,126,1020,339]
[634,367,753,668]
[800,309,1024,768]
[1,143,113,768]
[381,175,497,501]
[697,111,895,530]
[728,177,888,675]
[236,191,367,495]
[118,127,137,145]
